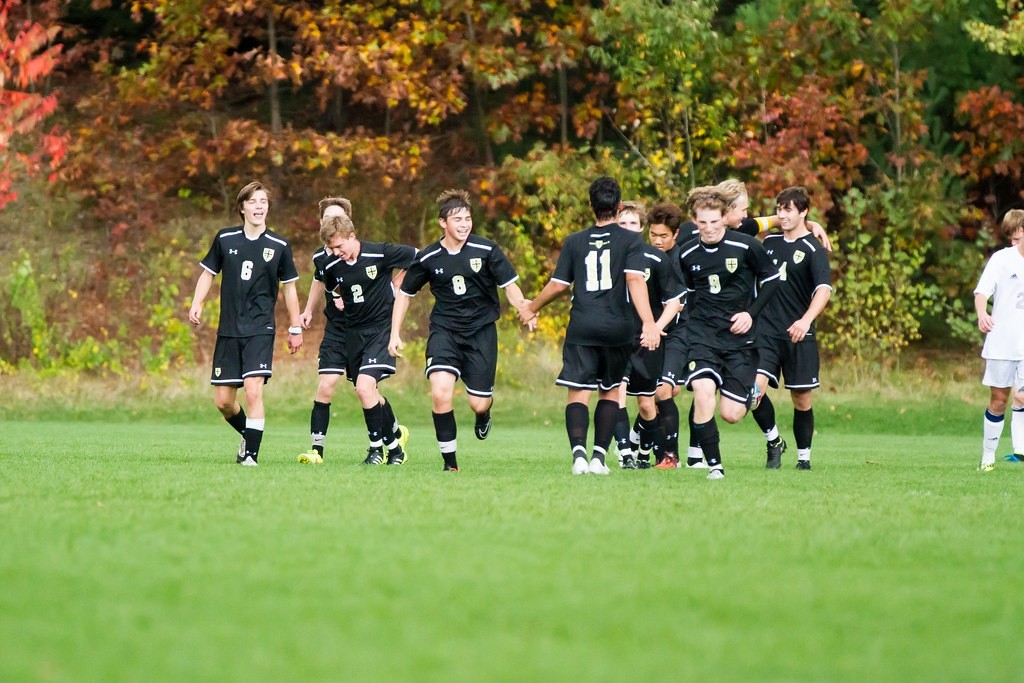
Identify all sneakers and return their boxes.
[797,459,811,470]
[474,410,491,440]
[974,462,994,475]
[385,425,409,462]
[588,458,610,473]
[298,449,322,463]
[654,452,681,468]
[444,463,458,472]
[687,460,707,469]
[636,458,650,469]
[623,459,636,469]
[363,450,383,465]
[386,452,405,466]
[572,456,589,473]
[751,381,761,410]
[707,466,724,480]
[766,437,786,469]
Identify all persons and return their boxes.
[300,200,409,463]
[972,209,1024,471]
[189,181,303,465]
[321,214,420,466]
[516,179,832,475]
[387,189,540,471]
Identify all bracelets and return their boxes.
[288,326,302,333]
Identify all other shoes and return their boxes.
[237,437,256,466]
